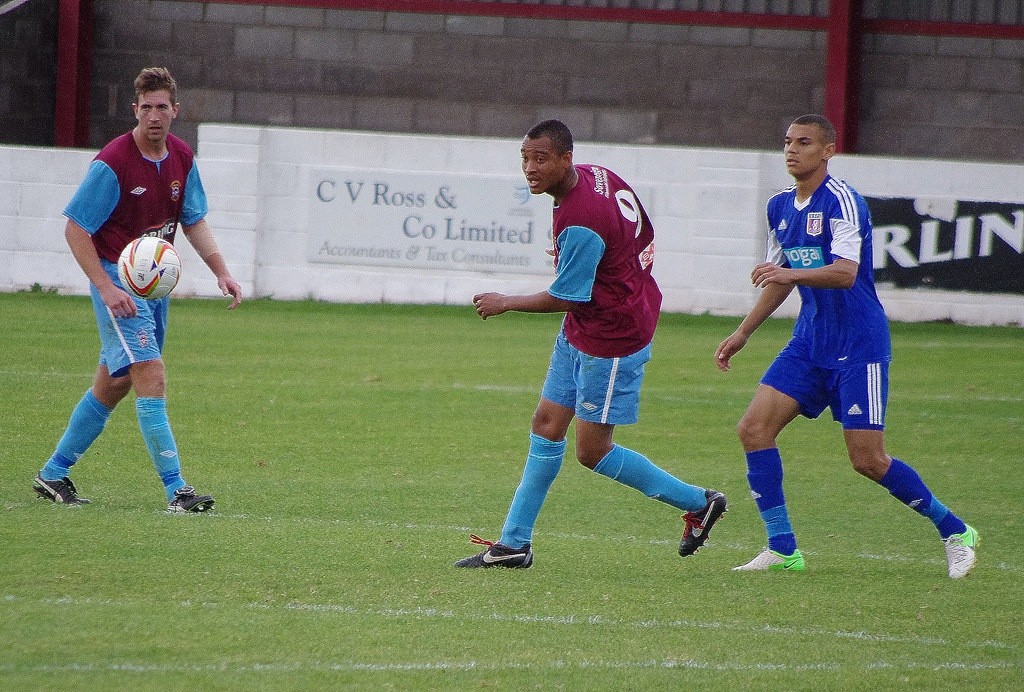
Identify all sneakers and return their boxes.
[33,470,91,506]
[942,523,979,579]
[455,534,533,570]
[169,485,214,513]
[678,489,729,558]
[731,548,805,572]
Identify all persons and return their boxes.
[32,67,242,513]
[715,114,980,579]
[453,120,728,571]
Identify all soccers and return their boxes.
[117,236,183,302]
